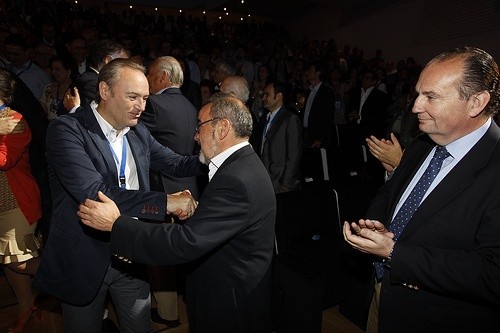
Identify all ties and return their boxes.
[375,146,452,280]
[263,115,270,135]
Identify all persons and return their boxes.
[0,1,423,333]
[341,47,500,333]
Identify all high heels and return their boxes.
[6,298,43,333]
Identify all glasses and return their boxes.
[195,118,219,131]
[34,51,50,57]
